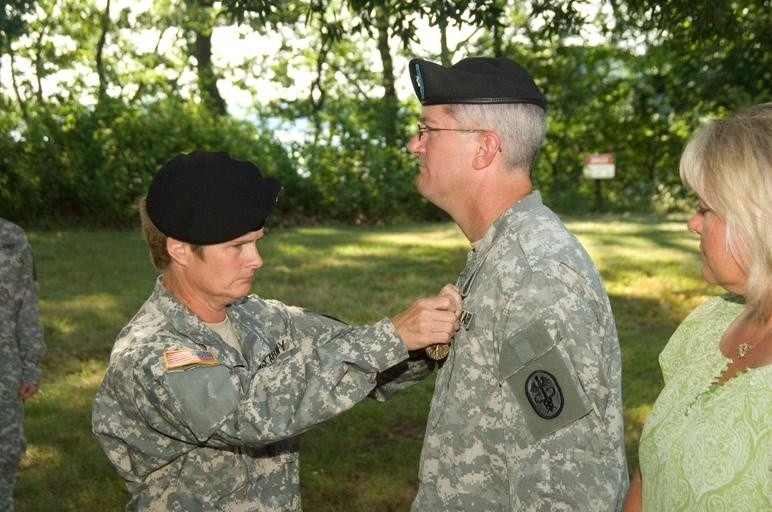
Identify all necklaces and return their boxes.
[736,342,752,360]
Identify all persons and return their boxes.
[622,101,772,512]
[0,216,48,511]
[406,56,631,512]
[91,150,463,512]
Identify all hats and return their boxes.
[145,150,283,248]
[408,52,550,111]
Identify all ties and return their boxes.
[417,119,503,157]
